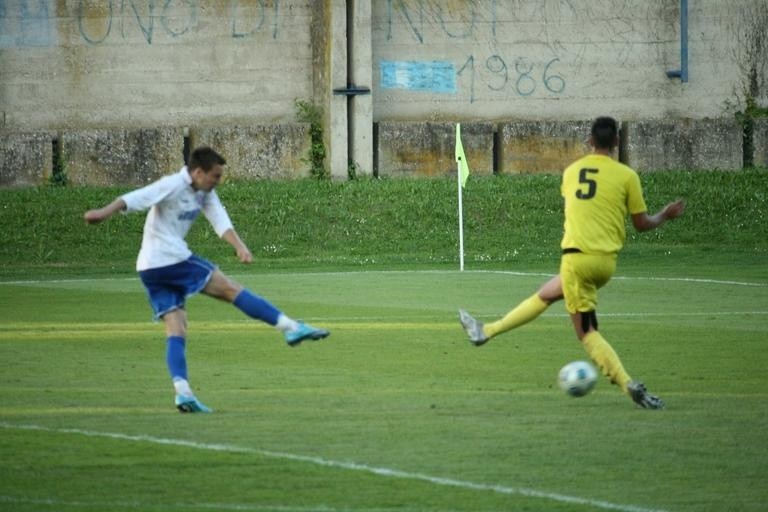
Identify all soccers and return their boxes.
[558,361,596,398]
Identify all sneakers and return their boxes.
[458,308,489,346]
[285,323,329,346]
[175,392,209,412]
[629,381,665,410]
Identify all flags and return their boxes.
[456,122,470,188]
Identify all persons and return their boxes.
[458,114,684,409]
[84,147,330,414]
